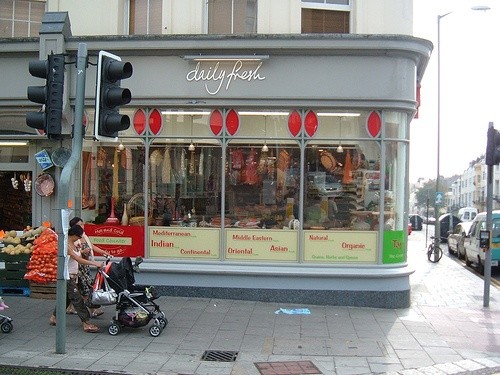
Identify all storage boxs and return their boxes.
[0,239,33,288]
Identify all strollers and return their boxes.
[0,297,14,333]
[97,255,169,337]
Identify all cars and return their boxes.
[308,173,344,200]
[446,222,473,259]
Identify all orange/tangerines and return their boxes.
[24,229,58,283]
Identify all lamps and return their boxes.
[260,115,269,152]
[0,140,31,147]
[119,131,124,151]
[189,115,196,152]
[336,115,344,153]
[181,50,271,63]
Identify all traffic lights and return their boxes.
[486,129,500,166]
[26,50,62,137]
[95,50,133,138]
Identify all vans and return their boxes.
[463,211,500,277]
[347,169,380,191]
[457,207,480,220]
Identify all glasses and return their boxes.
[76,234,82,239]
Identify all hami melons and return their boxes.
[1,225,46,255]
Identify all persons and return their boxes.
[0,296,8,310]
[328,197,338,221]
[66,216,113,317]
[49,224,100,331]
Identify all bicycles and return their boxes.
[427,236,443,263]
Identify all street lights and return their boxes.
[434,5,492,262]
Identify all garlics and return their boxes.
[11,178,18,189]
[23,179,31,191]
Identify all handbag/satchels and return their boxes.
[92,275,118,305]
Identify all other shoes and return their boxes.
[84,324,98,332]
[66,307,77,314]
[89,308,105,318]
[49,316,57,326]
